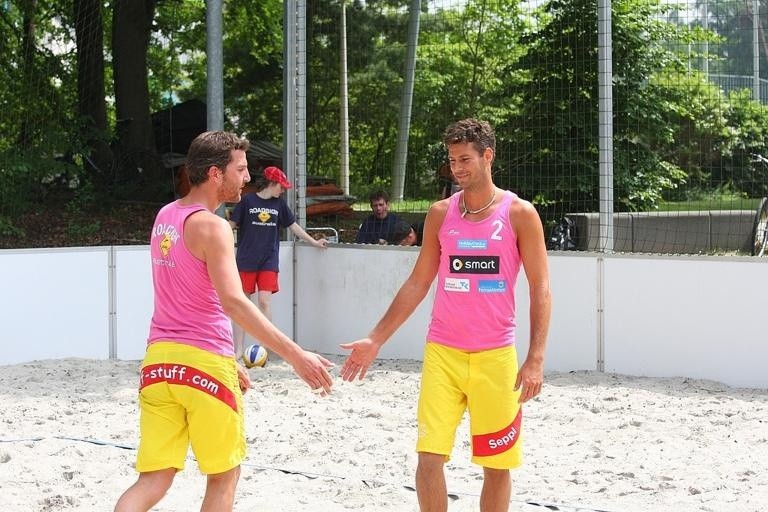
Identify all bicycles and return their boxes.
[747,151,768,258]
[549,217,575,251]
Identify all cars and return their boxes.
[659,150,767,198]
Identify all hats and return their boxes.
[263,165,295,191]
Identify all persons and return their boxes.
[355,191,400,245]
[338,117,551,511]
[385,221,423,246]
[114,129,336,510]
[228,166,329,360]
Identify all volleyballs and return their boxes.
[243,344,268,368]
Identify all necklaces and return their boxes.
[461,189,497,217]
